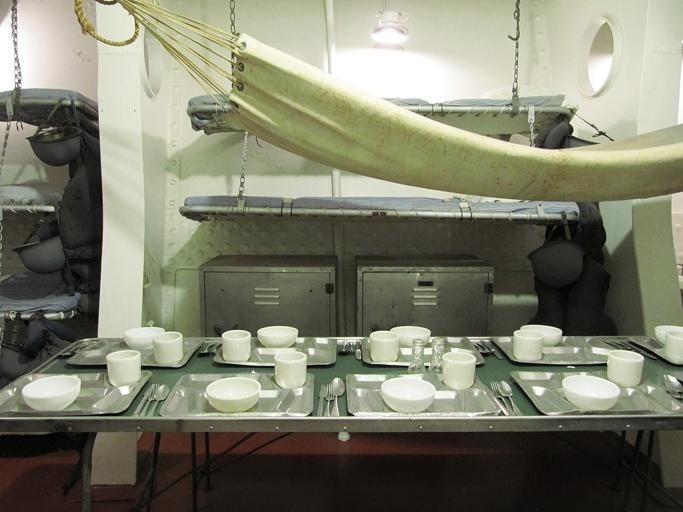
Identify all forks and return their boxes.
[490,380,510,421]
[323,383,334,418]
[137,385,159,417]
[349,341,354,355]
[662,377,682,400]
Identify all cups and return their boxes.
[511,330,543,363]
[663,330,682,360]
[220,329,252,362]
[605,349,646,387]
[153,329,185,365]
[106,350,142,388]
[369,330,398,363]
[441,351,476,390]
[273,350,306,389]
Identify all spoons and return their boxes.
[499,379,524,420]
[146,384,168,417]
[662,374,682,393]
[337,340,349,355]
[198,342,223,355]
[59,342,85,356]
[330,376,345,420]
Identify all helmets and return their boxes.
[0,342,34,380]
[26,128,82,166]
[527,241,583,288]
[11,235,65,274]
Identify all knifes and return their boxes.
[316,384,326,418]
[132,384,154,418]
[353,341,362,361]
[604,339,657,360]
[473,341,502,360]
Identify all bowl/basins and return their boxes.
[257,324,298,349]
[378,376,436,414]
[560,374,620,411]
[654,325,681,345]
[122,326,164,350]
[21,375,78,412]
[520,324,563,346]
[204,377,260,413]
[389,325,430,347]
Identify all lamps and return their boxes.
[364,2,414,51]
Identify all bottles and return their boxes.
[426,337,447,376]
[404,338,428,375]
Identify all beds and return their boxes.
[0,116,67,216]
[178,112,579,229]
[186,1,579,141]
[0,1,99,128]
[0,223,82,322]
